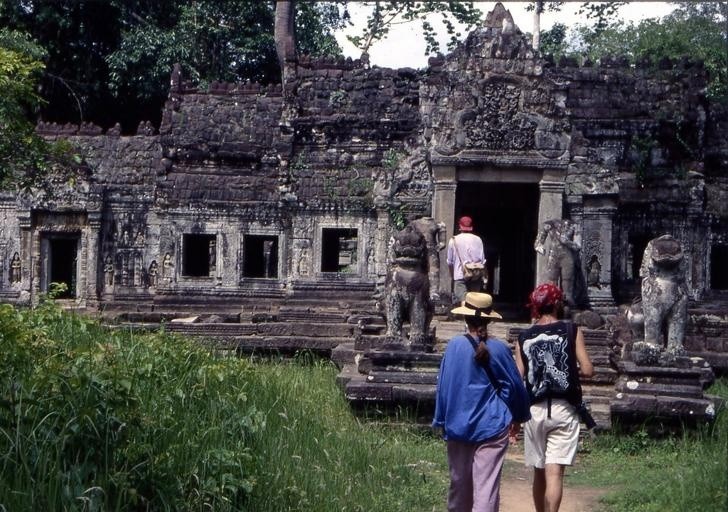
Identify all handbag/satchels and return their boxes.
[461,262,487,282]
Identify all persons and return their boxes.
[432,293,532,512]
[446,217,487,332]
[534,218,583,306]
[510,283,595,512]
[407,215,446,301]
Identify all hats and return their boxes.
[458,215,475,232]
[449,289,504,320]
[527,283,564,316]
